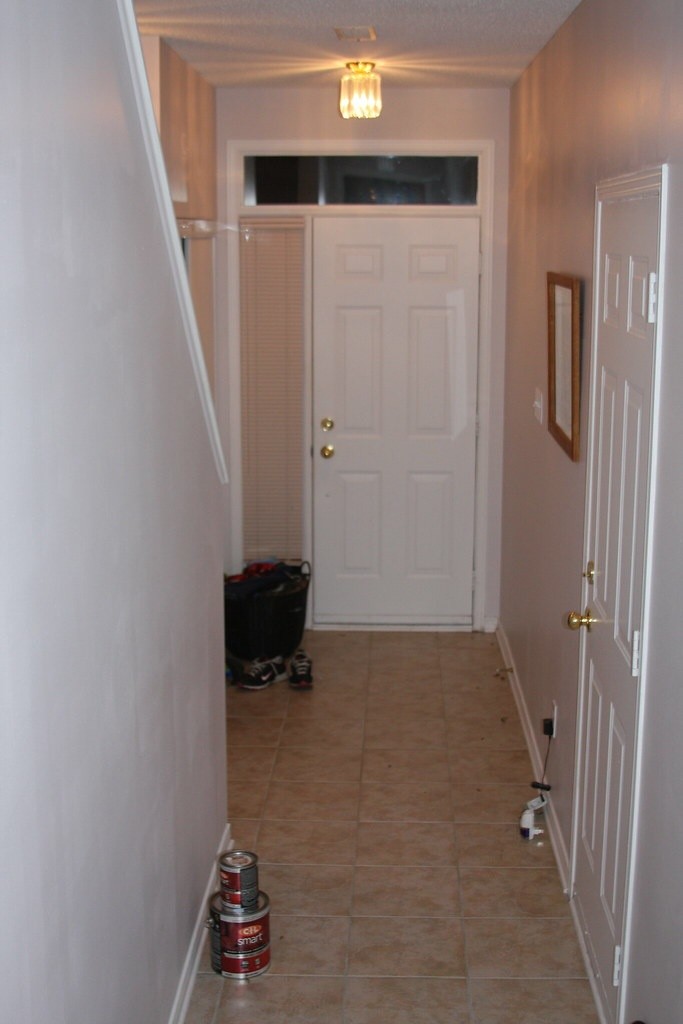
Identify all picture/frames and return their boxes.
[545,268,582,464]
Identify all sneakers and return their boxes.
[240,654,289,689]
[290,649,316,690]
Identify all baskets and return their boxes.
[224,555,312,670]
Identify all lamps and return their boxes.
[338,62,384,120]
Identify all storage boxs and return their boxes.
[225,560,312,661]
